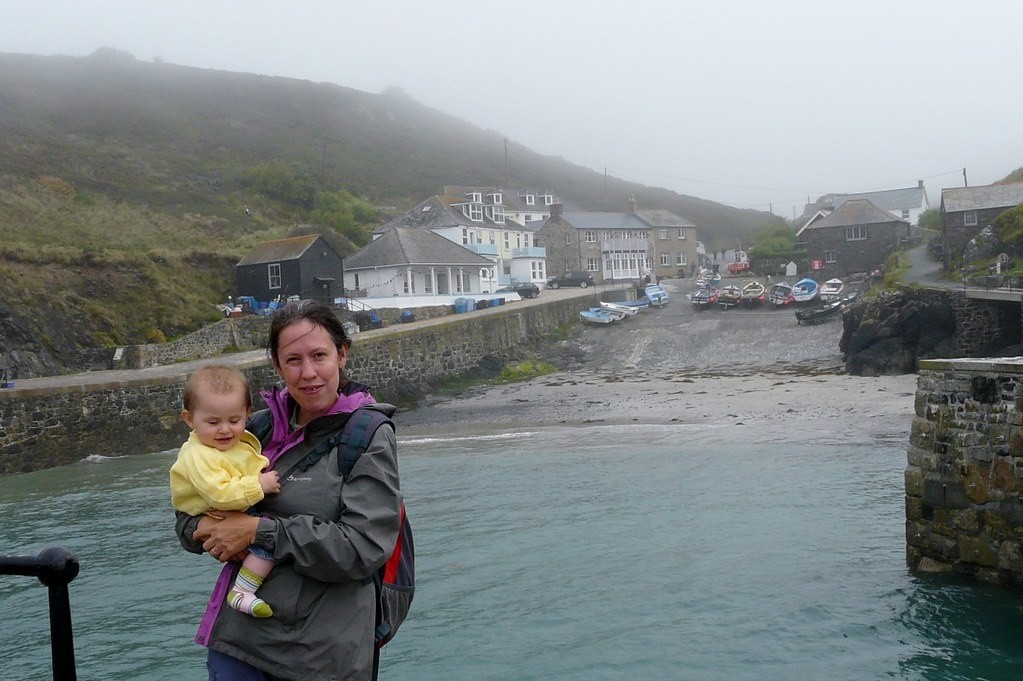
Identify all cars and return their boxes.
[496,282,540,299]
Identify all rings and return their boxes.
[212,548,219,556]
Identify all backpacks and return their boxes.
[245,402,416,648]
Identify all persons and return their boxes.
[169,366,287,622]
[171,297,404,681]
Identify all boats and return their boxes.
[684,259,861,325]
[579,284,669,326]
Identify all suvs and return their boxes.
[546,270,594,289]
